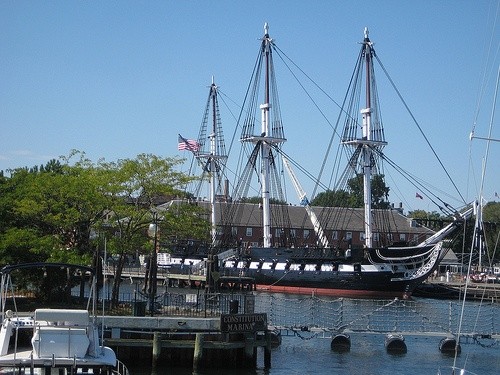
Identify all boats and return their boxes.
[0,258,130,375]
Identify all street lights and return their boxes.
[148,211,159,299]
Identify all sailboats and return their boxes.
[152,20,478,301]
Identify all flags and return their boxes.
[416,193,423,200]
[178,136,199,152]
